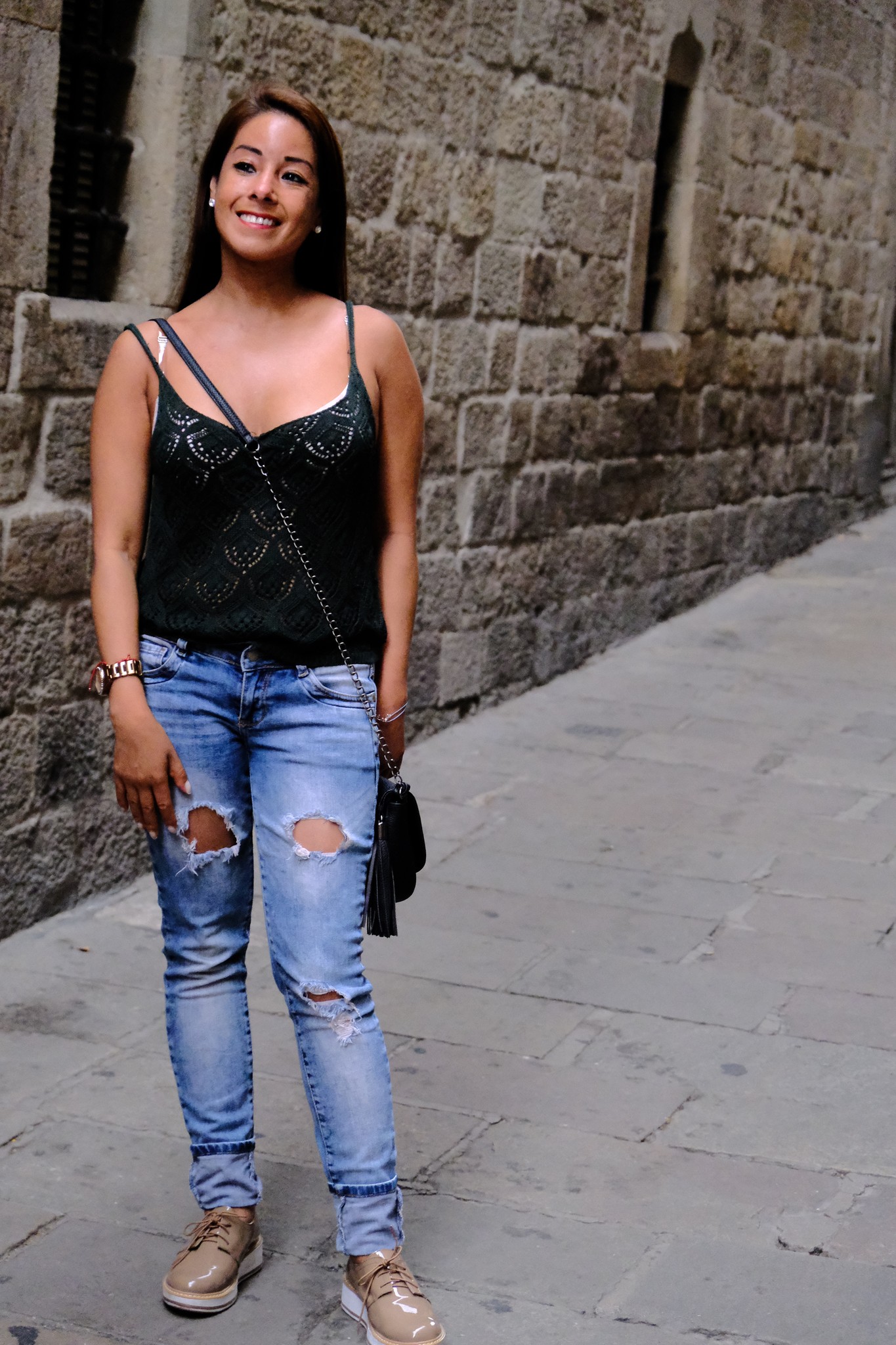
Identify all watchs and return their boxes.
[89,654,143,696]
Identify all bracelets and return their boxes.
[376,702,408,722]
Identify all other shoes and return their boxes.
[162,1206,264,1313]
[342,1246,446,1345]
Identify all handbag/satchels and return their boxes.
[361,776,428,941]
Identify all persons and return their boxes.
[90,90,446,1345]
